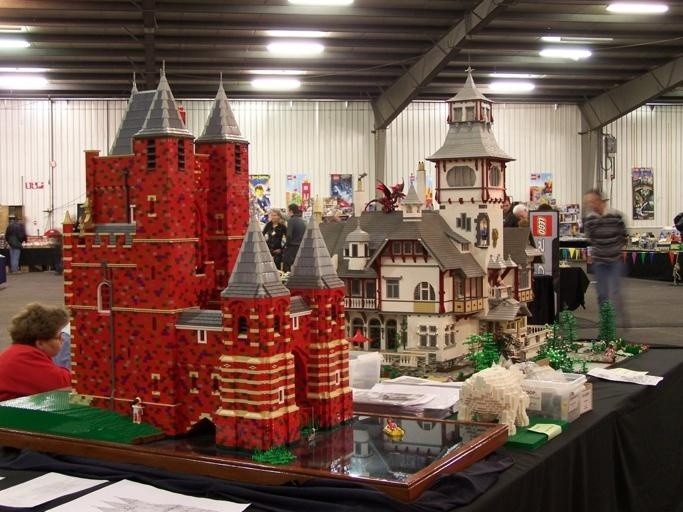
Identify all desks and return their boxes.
[0,336,683,512]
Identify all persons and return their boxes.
[605,344,614,356]
[584,189,629,333]
[1,303,72,401]
[503,196,553,227]
[387,418,398,431]
[132,399,144,424]
[4,216,64,275]
[262,203,307,273]
